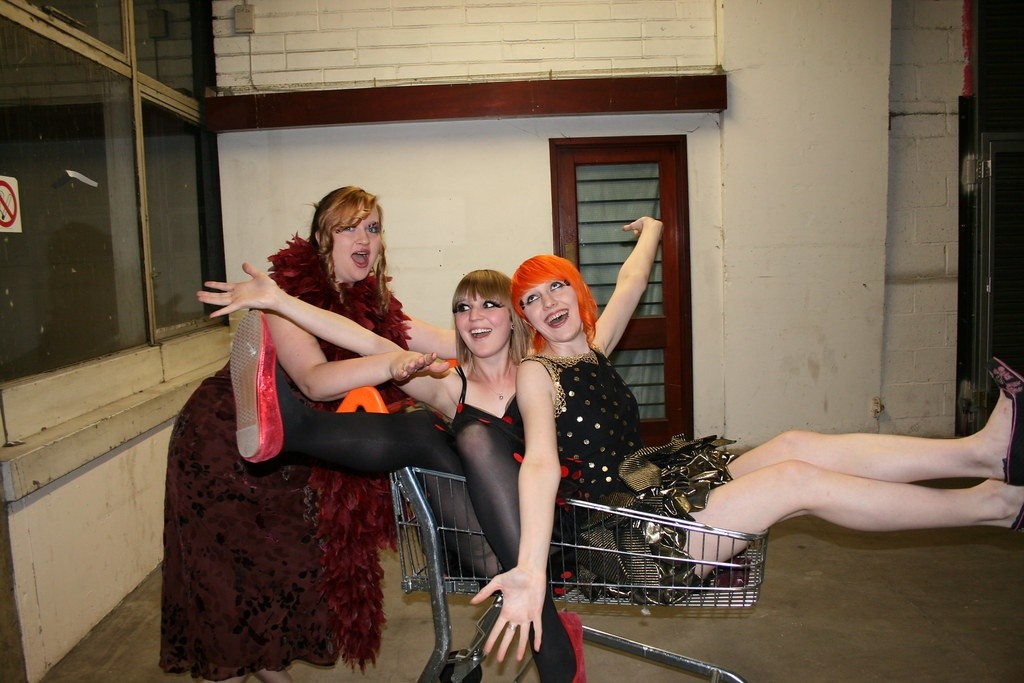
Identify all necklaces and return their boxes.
[471,360,511,399]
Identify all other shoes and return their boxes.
[1010,502,1024,534]
[230,310,284,463]
[988,356,1024,486]
[557,611,586,683]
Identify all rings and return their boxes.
[508,624,516,631]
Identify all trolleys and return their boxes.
[336,385,772,683]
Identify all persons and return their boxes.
[160,183,458,683]
[193,217,1024,683]
